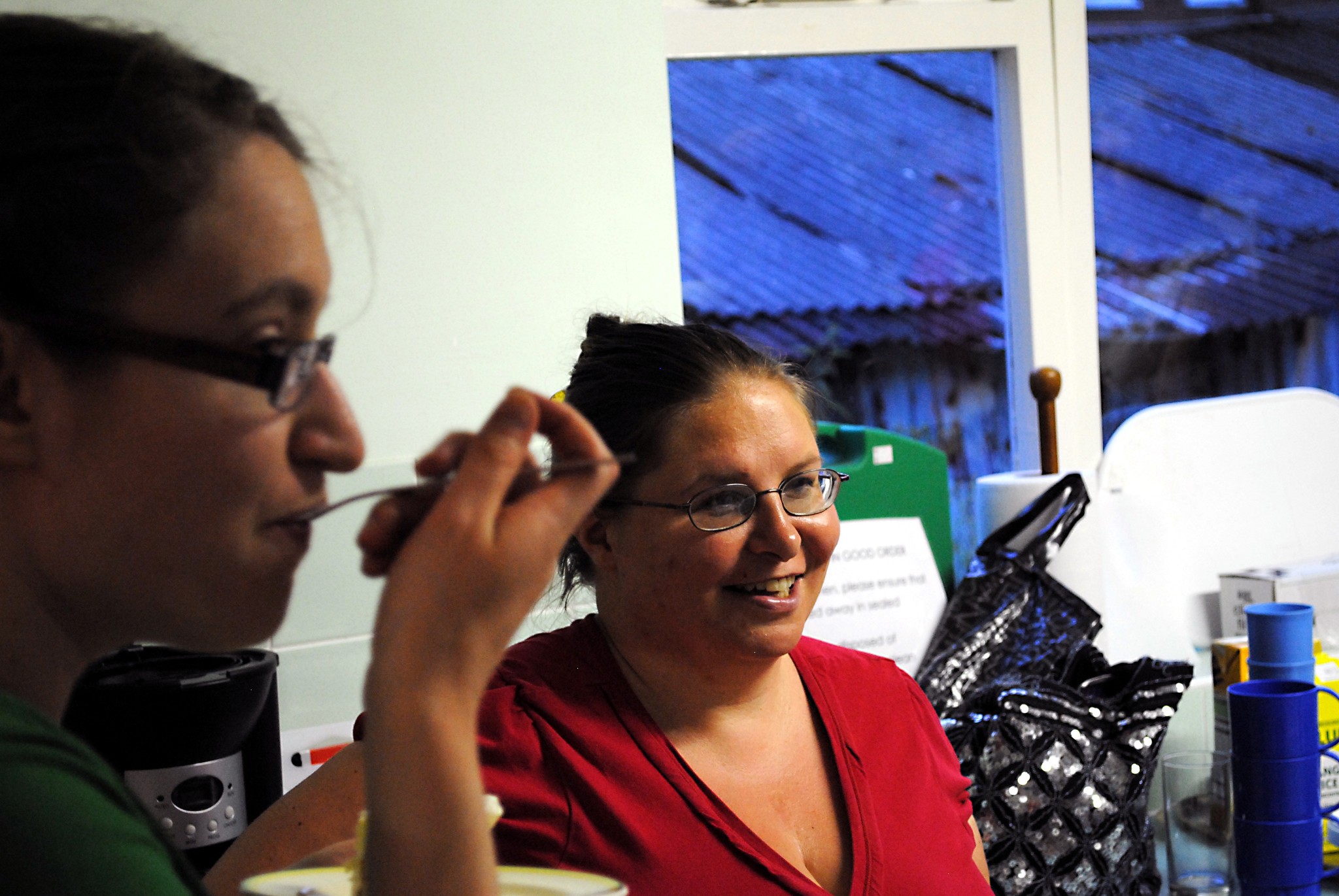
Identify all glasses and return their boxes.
[630,468,851,533]
[111,310,336,414]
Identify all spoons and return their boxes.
[277,455,638,524]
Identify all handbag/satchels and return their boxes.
[915,471,1196,895]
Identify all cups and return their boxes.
[1163,601,1338,896]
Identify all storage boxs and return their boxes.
[1206,553,1339,871]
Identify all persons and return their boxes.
[2,10,618,896]
[476,314,998,896]
